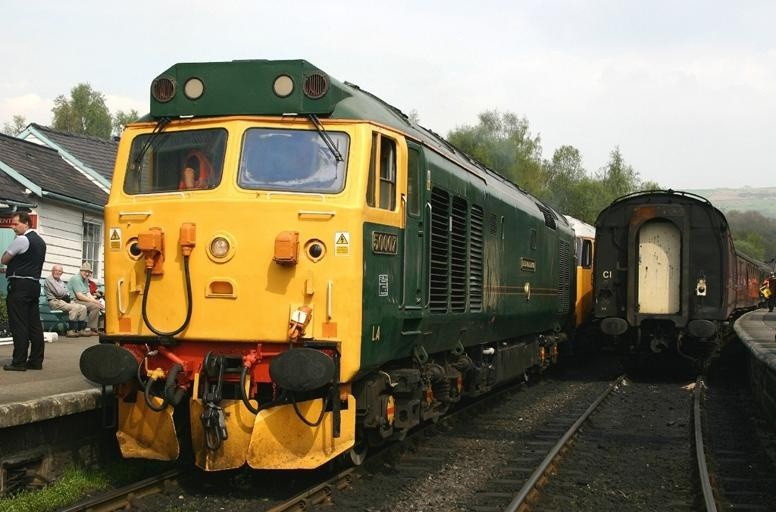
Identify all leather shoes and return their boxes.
[3,363,42,371]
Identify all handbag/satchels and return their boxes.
[62,294,70,303]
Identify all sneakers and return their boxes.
[66,329,99,337]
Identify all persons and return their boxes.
[179,148,215,190]
[1,211,47,371]
[45,262,105,338]
[760,272,776,313]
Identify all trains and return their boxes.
[592,188,776,379]
[76,59,596,494]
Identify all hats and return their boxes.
[80,261,92,272]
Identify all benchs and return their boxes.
[48,311,105,334]
